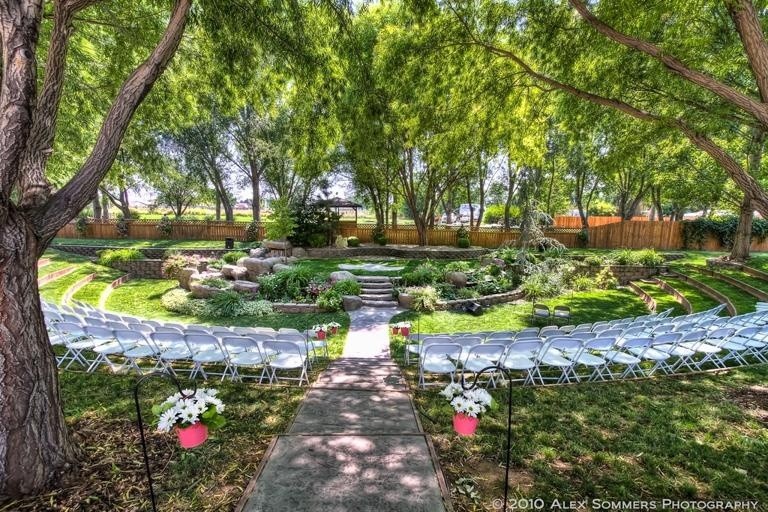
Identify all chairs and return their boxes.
[40,300,331,390]
[406,303,767,396]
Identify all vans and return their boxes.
[234,204,239,209]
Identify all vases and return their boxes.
[172,421,208,450]
[452,412,478,437]
[392,329,398,334]
[332,328,337,335]
[400,327,409,336]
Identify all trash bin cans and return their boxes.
[225,238,234,249]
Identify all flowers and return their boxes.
[440,382,501,421]
[152,390,227,433]
[328,322,342,328]
[388,322,409,329]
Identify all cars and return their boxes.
[570,209,588,217]
[434,203,481,225]
